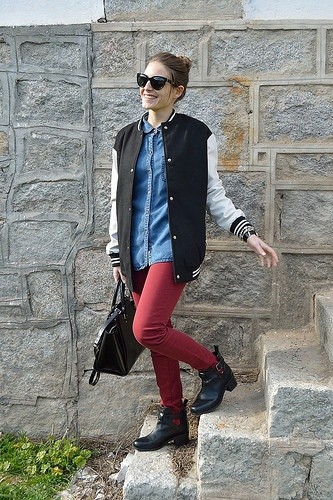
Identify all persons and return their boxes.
[106,53,280,452]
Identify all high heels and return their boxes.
[133,399,190,451]
[190,345,237,414]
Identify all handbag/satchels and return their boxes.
[88,278,147,385]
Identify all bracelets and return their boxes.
[242,230,259,243]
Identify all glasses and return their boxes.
[136,72,177,90]
